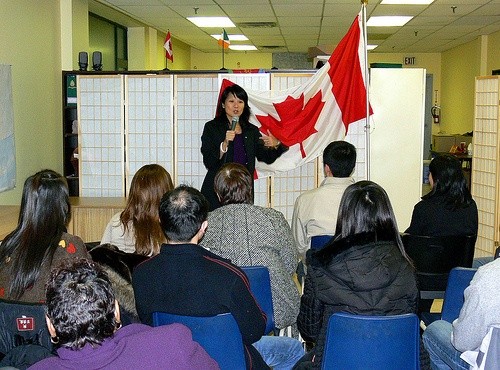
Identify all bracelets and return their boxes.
[219,142,229,153]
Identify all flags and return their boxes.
[164,31,174,64]
[213,4,374,181]
[218,29,231,50]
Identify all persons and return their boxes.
[25,258,221,370]
[420,256,500,370]
[291,179,431,369]
[291,141,358,268]
[398,153,479,317]
[0,168,94,306]
[132,183,308,369]
[195,162,301,342]
[99,163,178,255]
[198,84,288,215]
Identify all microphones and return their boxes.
[232,115,239,130]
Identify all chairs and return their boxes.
[423,266,479,327]
[240,265,280,337]
[321,312,422,369]
[0,299,52,356]
[153,310,247,370]
